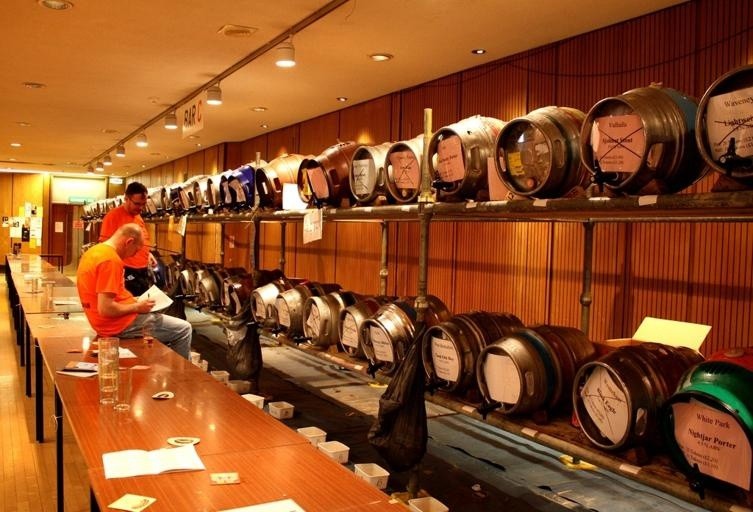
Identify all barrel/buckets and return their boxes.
[85,67,753,218]
[144,247,752,507]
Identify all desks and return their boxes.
[4,254,201,444]
[37,337,311,512]
[87,443,414,512]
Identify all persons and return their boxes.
[74,182,193,356]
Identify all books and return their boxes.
[55,360,99,378]
[101,447,205,480]
[119,345,137,359]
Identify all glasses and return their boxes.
[129,198,147,205]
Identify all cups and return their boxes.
[94,335,121,405]
[30,278,55,301]
[142,324,154,346]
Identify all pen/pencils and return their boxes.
[148,294,150,301]
[63,368,97,372]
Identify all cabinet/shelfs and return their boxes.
[419,108,753,512]
[138,152,590,490]
